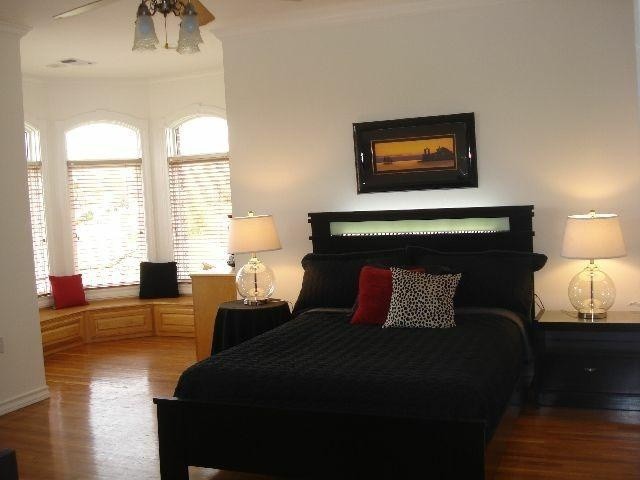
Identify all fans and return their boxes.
[52,0,215,26]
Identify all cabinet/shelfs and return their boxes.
[39,296,196,356]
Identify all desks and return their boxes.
[188,273,237,361]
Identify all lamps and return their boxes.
[226,210,283,306]
[131,0,216,55]
[560,210,627,318]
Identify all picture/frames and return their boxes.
[351,112,479,195]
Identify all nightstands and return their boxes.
[211,298,292,356]
[531,310,640,411]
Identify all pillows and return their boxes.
[138,261,180,300]
[48,274,89,310]
[289,245,548,329]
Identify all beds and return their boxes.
[153,206,534,480]
